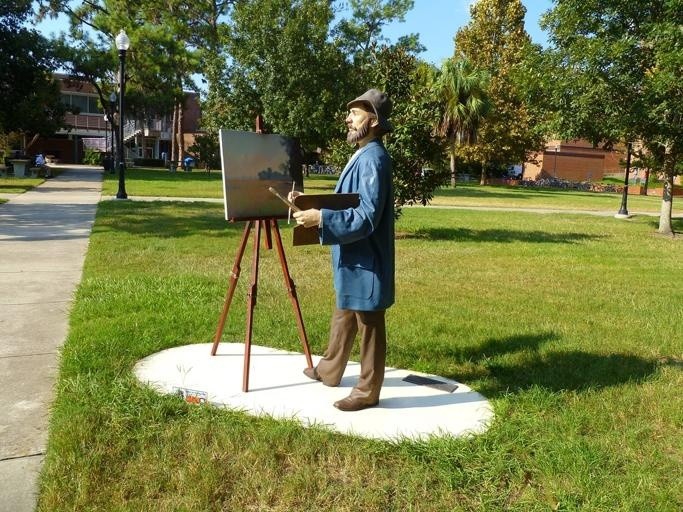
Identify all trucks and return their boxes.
[505,163,523,179]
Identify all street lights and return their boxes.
[614,126,636,219]
[114,26,129,199]
[554,144,558,180]
[103,89,117,175]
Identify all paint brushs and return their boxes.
[269,181,322,237]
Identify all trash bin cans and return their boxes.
[423,170,435,176]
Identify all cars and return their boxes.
[36,154,45,165]
[305,159,340,175]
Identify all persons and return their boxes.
[287,90,396,412]
[184,155,193,171]
[36,153,54,178]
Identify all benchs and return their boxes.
[28,166,40,177]
[170,159,211,175]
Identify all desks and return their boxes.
[10,160,31,177]
